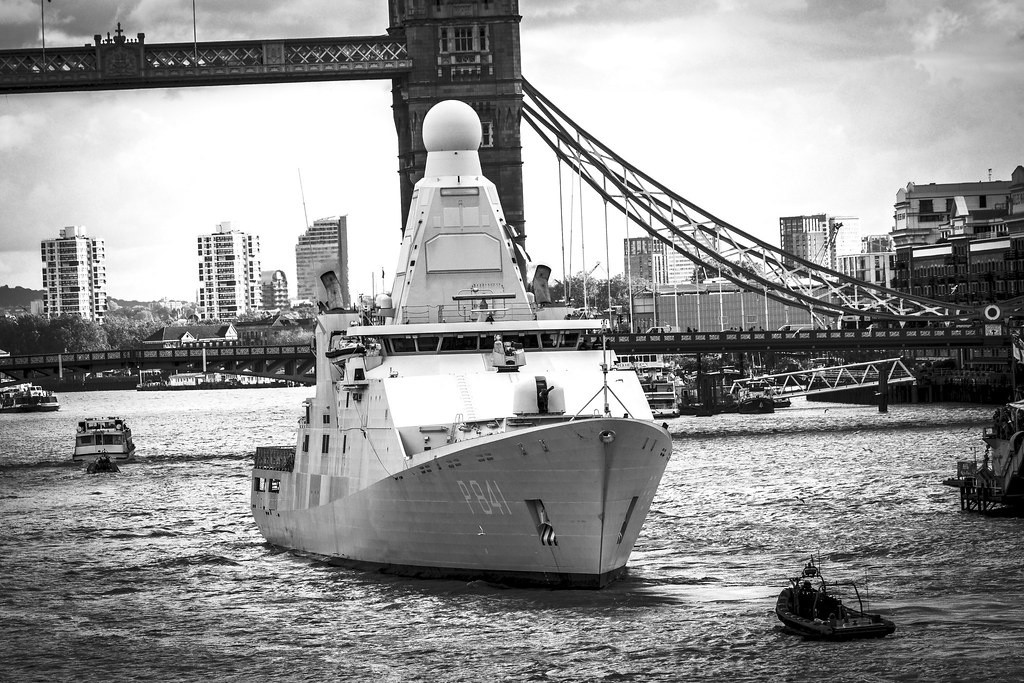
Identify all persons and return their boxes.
[563,314,572,320]
[486,315,495,323]
[588,321,955,333]
[796,580,817,620]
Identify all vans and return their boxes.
[773,323,815,338]
[644,325,680,341]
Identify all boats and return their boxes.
[0,378,61,413]
[639,366,792,419]
[774,550,896,643]
[249,99,674,589]
[86,448,122,474]
[72,416,137,462]
